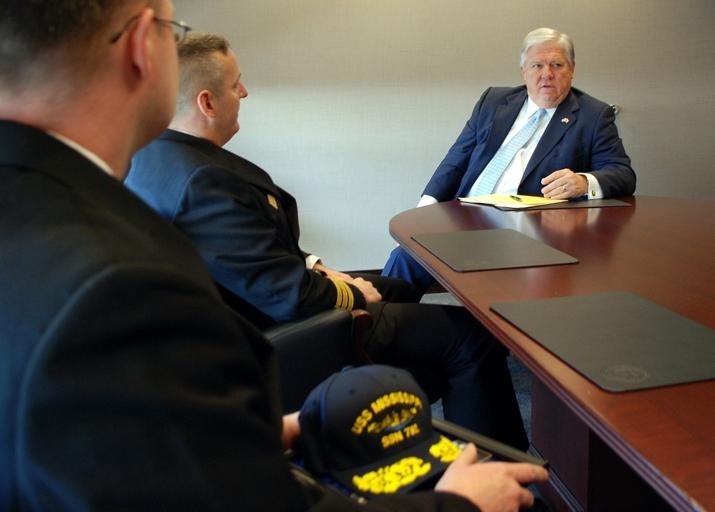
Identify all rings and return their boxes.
[563,185,567,191]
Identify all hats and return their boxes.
[297,362,464,500]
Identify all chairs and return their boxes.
[254,307,374,420]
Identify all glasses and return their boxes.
[108,13,193,45]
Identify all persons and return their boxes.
[119,33,533,489]
[378,28,636,305]
[0,0,550,512]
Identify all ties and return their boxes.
[471,106,548,197]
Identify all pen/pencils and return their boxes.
[510,195,522,202]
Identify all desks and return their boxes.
[389,195,715,512]
